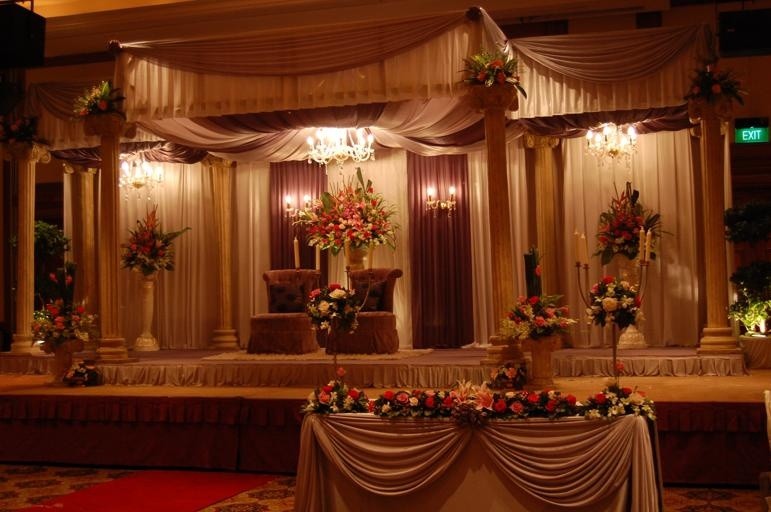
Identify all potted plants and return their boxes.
[723,200,771,370]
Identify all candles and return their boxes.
[450,192,453,202]
[294,235,375,272]
[306,200,309,208]
[428,190,431,201]
[286,201,290,211]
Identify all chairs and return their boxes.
[327,268,402,355]
[248,269,321,354]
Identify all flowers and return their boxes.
[71,79,126,125]
[306,284,360,337]
[25,259,103,386]
[291,167,400,253]
[0,113,40,144]
[453,40,528,100]
[490,180,676,341]
[119,203,192,276]
[683,63,749,107]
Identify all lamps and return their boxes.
[586,121,640,163]
[117,161,164,202]
[306,127,376,178]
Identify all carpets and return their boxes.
[199,347,434,360]
